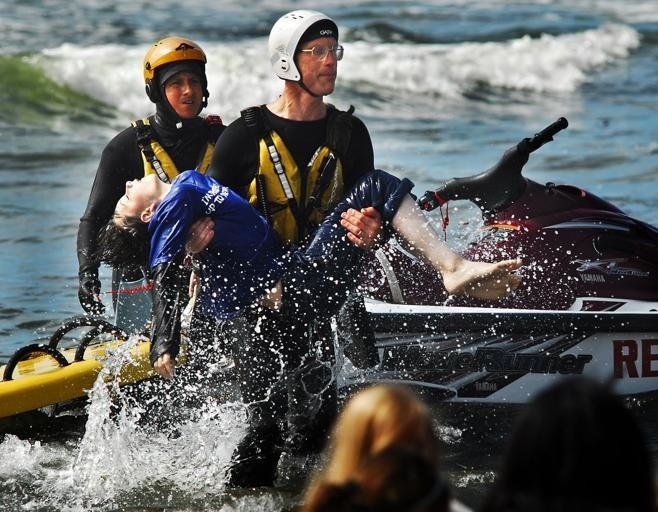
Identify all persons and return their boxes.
[479,375,658,512]
[178,10,383,494]
[76,36,230,340]
[297,385,473,512]
[104,171,525,380]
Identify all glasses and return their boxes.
[297,43,344,62]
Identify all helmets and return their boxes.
[143,36,207,103]
[269,9,338,82]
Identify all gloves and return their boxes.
[78,272,105,315]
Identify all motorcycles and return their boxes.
[355,115,658,401]
